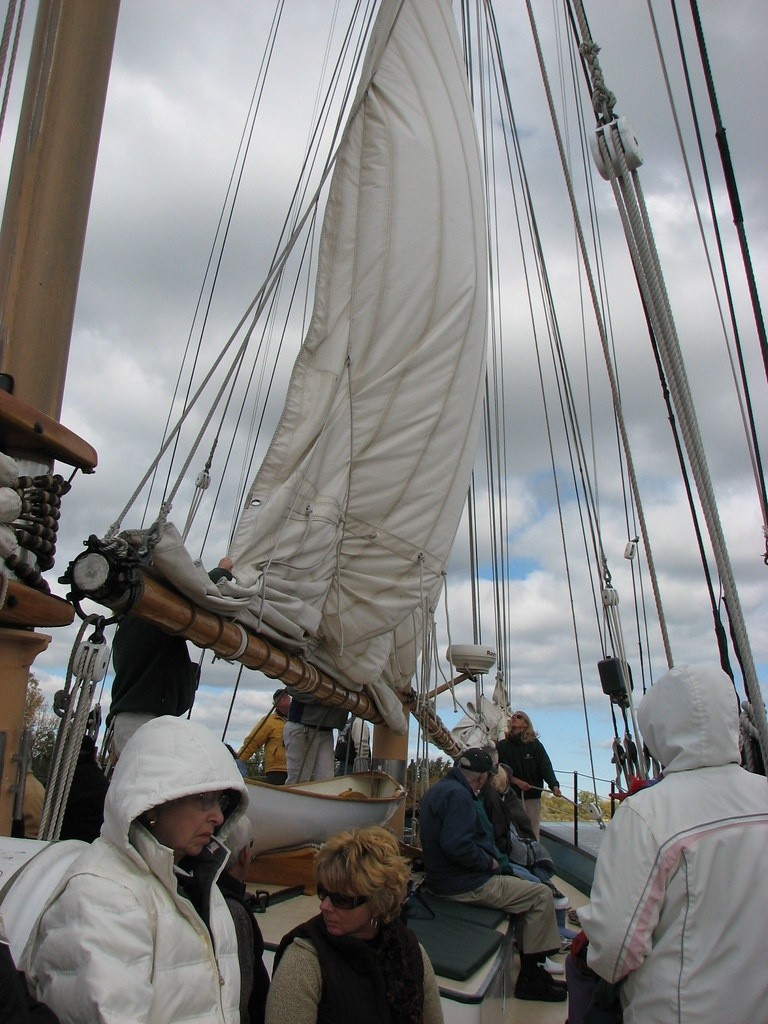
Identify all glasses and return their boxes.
[191,793,231,813]
[512,713,522,719]
[316,881,370,909]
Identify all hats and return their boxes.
[458,747,500,777]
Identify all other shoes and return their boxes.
[514,965,568,1003]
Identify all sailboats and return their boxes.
[0,3,767,1024]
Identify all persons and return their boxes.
[572,660,767,1022]
[0,557,570,1023]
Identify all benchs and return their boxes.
[394,879,515,1024]
